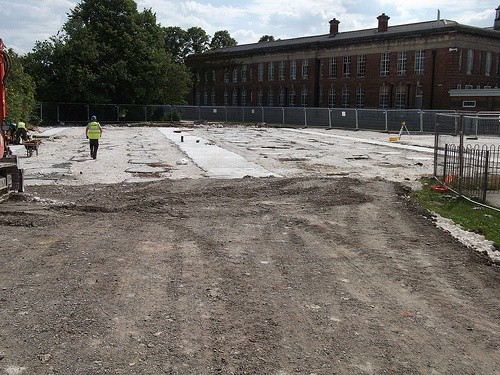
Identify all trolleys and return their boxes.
[22,139,43,157]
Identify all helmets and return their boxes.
[91,115,96,121]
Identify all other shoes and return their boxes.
[91,154,97,159]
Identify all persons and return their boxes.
[85,116,103,159]
[15,121,28,142]
